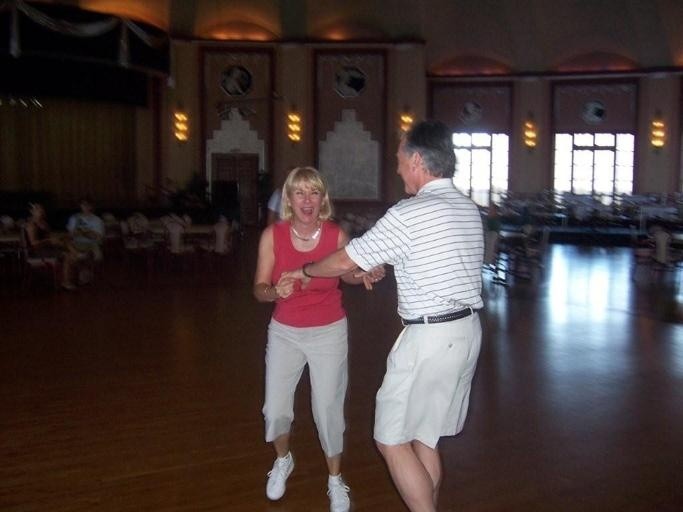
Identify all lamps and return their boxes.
[651,110,665,147]
[174,101,189,147]
[287,106,302,148]
[525,111,536,148]
[401,103,415,132]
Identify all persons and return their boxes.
[254,167,386,511]
[279,121,486,511]
[23,196,105,290]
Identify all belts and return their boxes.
[401,307,481,325]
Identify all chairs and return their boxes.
[0,212,239,292]
[629,223,682,284]
[483,225,549,286]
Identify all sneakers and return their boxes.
[326,473,350,512]
[265,452,295,500]
